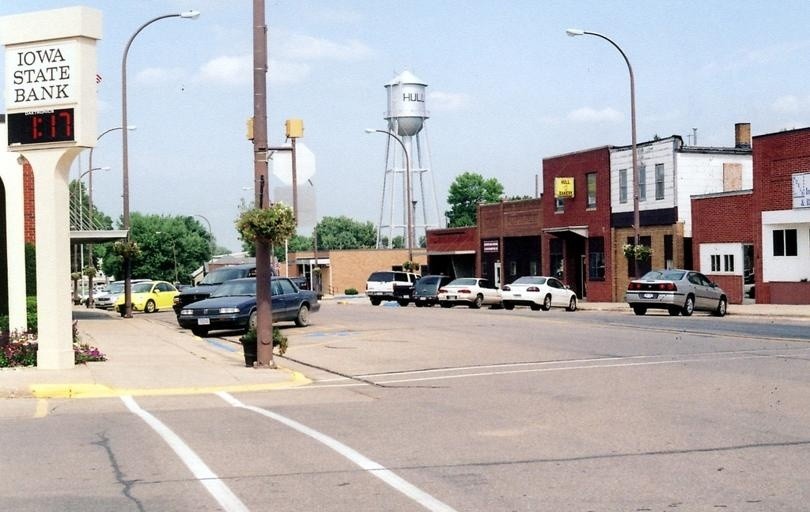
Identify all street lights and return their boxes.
[74,121,133,304]
[362,126,419,267]
[564,26,646,273]
[120,7,202,318]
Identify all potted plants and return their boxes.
[239,327,288,368]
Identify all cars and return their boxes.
[623,268,730,319]
[502,275,579,312]
[367,269,503,309]
[82,274,180,317]
[173,262,317,337]
